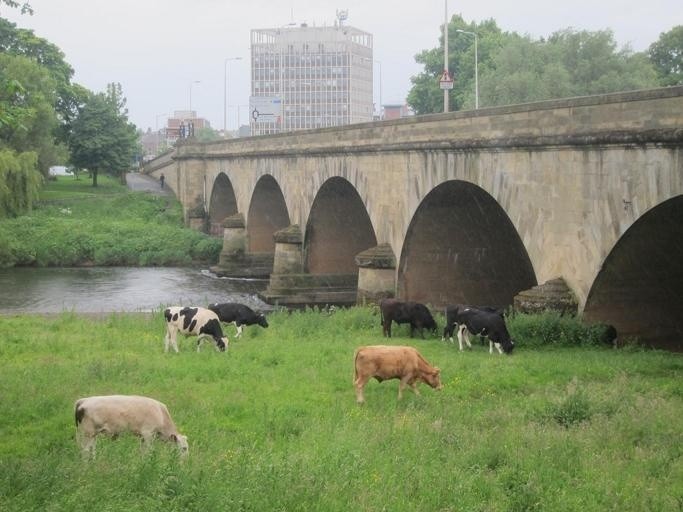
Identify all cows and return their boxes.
[442,303,505,346]
[208,303,268,342]
[353,345,442,406]
[457,307,515,355]
[380,299,439,339]
[74,395,189,464]
[163,307,229,354]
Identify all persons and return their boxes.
[160,173,164,188]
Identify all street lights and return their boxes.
[456,30,479,109]
[224,57,243,129]
[190,80,200,111]
